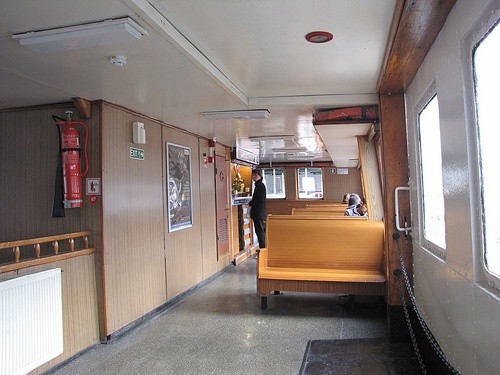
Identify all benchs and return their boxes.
[256,214,387,310]
[292,204,348,215]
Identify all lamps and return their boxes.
[11,17,149,54]
[200,111,271,121]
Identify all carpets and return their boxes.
[298,336,397,375]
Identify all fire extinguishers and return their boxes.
[52,109,83,218]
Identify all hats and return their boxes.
[252,169,262,176]
[348,194,361,206]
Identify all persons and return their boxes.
[341,192,367,217]
[249,168,267,248]
